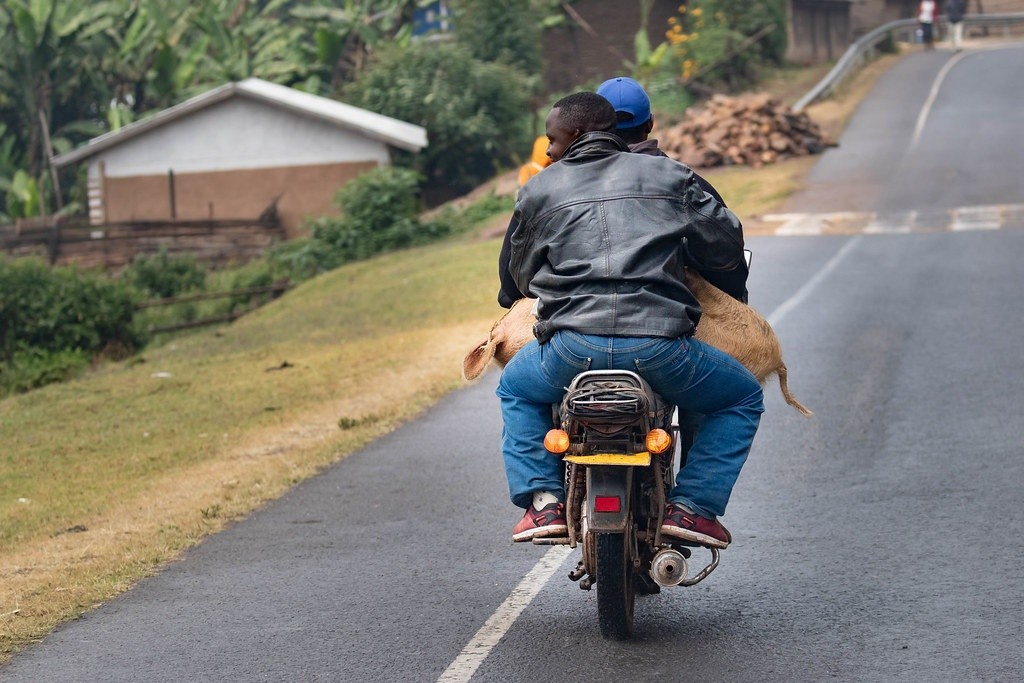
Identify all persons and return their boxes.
[551,77,706,471]
[497,92,764,549]
[917,0,939,53]
[519,135,554,188]
[942,0,969,52]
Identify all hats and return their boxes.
[596,76,656,133]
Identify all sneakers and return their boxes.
[511,501,569,542]
[662,502,729,550]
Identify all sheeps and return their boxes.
[465,266,816,421]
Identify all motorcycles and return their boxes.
[532,245,755,641]
